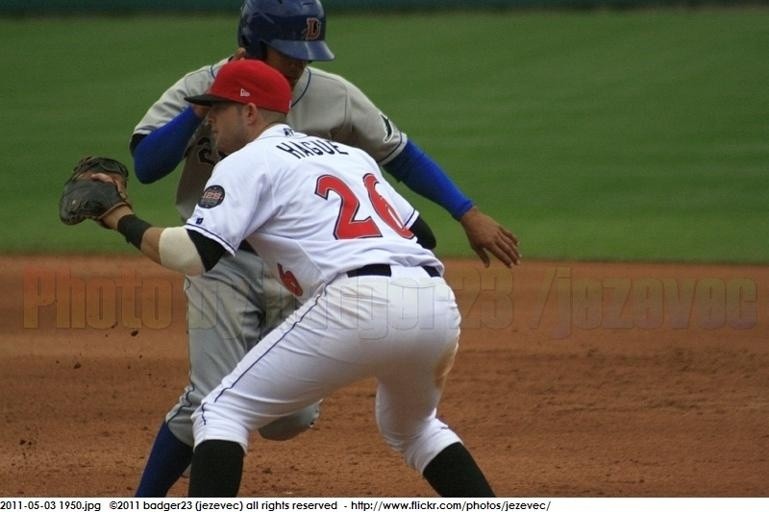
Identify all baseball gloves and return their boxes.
[59,155,132,226]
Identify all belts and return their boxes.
[344,261,442,279]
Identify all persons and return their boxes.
[130,1,522,498]
[55,59,498,497]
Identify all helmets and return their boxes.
[233,0,338,63]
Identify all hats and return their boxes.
[183,60,293,114]
